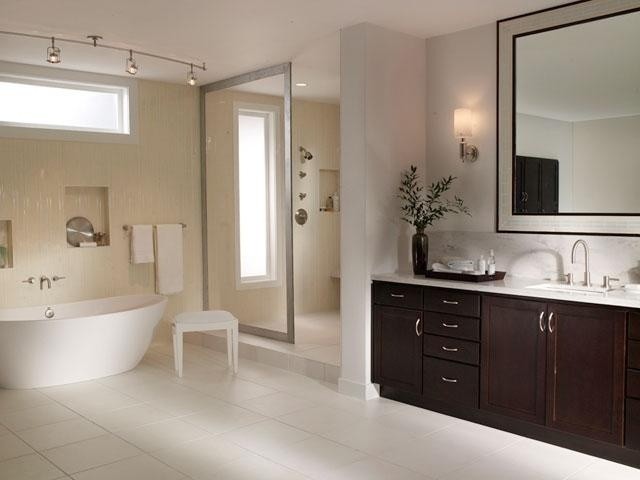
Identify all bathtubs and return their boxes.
[0,294,170,389]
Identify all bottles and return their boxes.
[478,254,486,276]
[487,248,495,277]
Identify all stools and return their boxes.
[171,310,239,376]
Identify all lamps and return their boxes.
[1,32,206,87]
[301,146,313,160]
[452,109,480,163]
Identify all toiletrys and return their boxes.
[476,254,485,274]
[489,249,496,275]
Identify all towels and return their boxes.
[131,225,154,264]
[154,224,184,295]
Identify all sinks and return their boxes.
[525,284,618,293]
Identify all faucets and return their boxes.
[40,277,51,290]
[571,239,592,288]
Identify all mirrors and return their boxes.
[496,0,640,238]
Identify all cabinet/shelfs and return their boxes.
[371,273,481,425]
[515,155,559,213]
[483,275,638,468]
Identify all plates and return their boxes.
[67,215,95,248]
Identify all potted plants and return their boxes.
[396,165,471,275]
